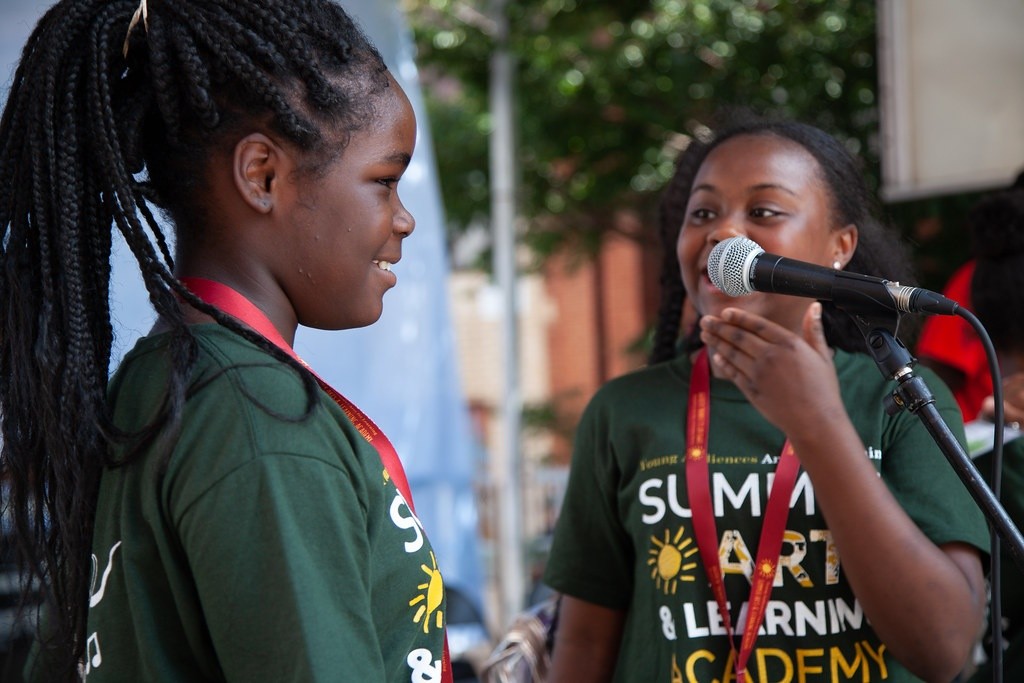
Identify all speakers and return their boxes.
[874,1,1023,202]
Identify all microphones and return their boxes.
[707,236,960,316]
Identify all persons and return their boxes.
[542,122,991,683]
[1,1,452,683]
